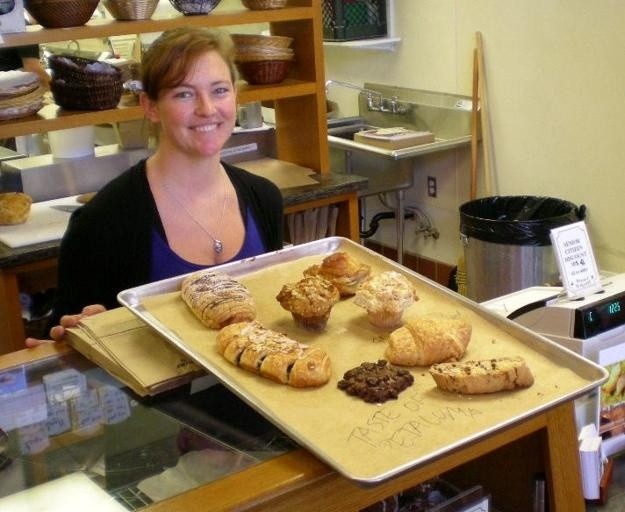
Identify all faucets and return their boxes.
[326,79,406,116]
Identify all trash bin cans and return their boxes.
[459,196,586,304]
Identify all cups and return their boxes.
[238,101,263,129]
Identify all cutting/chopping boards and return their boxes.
[0,190,100,250]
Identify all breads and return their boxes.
[303,252,371,298]
[429,358,534,394]
[180,268,257,329]
[352,271,420,328]
[0,192,32,225]
[215,321,331,387]
[384,311,471,365]
[275,276,339,331]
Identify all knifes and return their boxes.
[47,203,83,214]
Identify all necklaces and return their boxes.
[154,163,230,253]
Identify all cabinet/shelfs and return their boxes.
[0,0,367,351]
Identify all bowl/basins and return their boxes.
[47,124,96,158]
[113,118,151,150]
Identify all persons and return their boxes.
[0,18,51,152]
[25,24,284,348]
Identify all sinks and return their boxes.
[328,123,472,160]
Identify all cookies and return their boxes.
[338,359,414,403]
